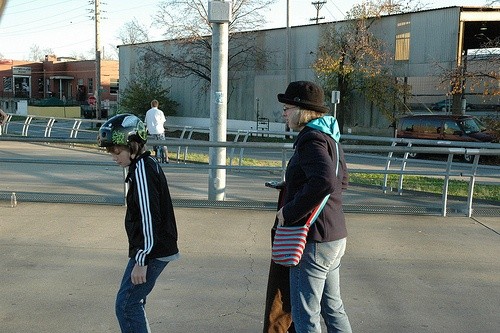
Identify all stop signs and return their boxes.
[88,96,96,105]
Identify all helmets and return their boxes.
[96,113,148,148]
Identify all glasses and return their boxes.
[282,104,297,112]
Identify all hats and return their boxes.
[277,80,331,113]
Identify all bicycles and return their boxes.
[146,127,170,164]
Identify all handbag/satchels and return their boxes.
[270,225,309,267]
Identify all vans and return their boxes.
[396,112,500,163]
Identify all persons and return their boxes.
[263,180,298,333]
[97,113,183,333]
[272,81,353,333]
[0,108,8,125]
[146,99,171,164]
